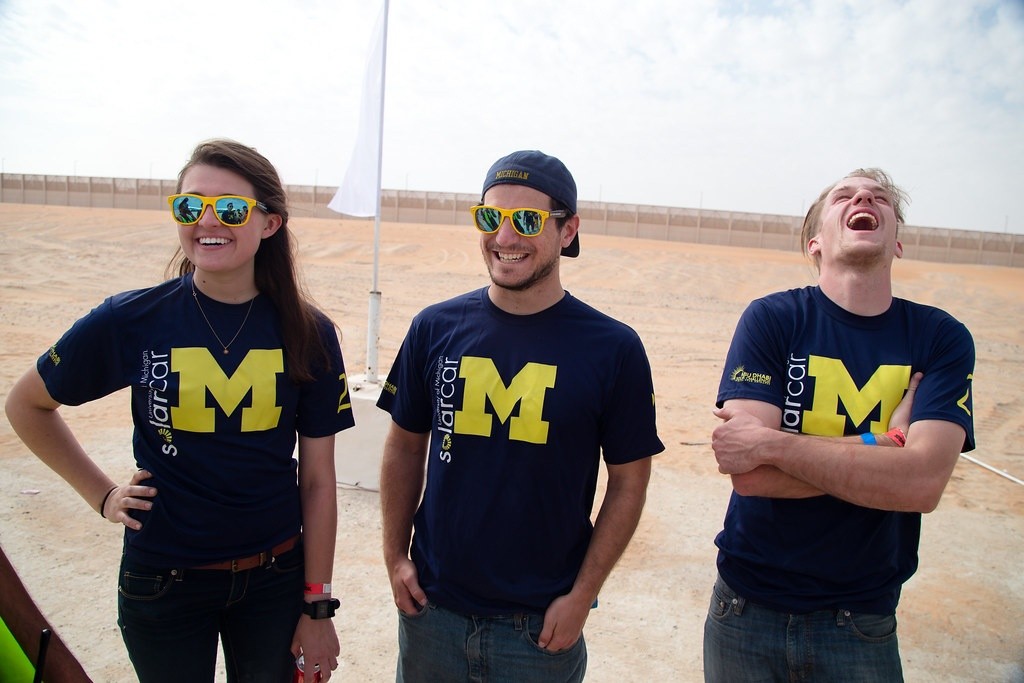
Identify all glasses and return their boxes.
[470,203,566,237]
[168,193,271,227]
[228,206,233,208]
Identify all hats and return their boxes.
[481,150,580,258]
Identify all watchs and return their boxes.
[302,598,340,620]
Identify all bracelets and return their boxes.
[882,427,906,447]
[304,581,331,594]
[100,486,117,518]
[861,432,877,445]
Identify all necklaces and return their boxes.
[191,277,255,354]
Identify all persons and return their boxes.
[703,167,976,683]
[175,198,203,224]
[474,208,501,232]
[513,211,542,235]
[375,150,666,683]
[221,203,248,224]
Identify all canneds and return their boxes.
[293,653,322,683]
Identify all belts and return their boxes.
[191,535,300,572]
